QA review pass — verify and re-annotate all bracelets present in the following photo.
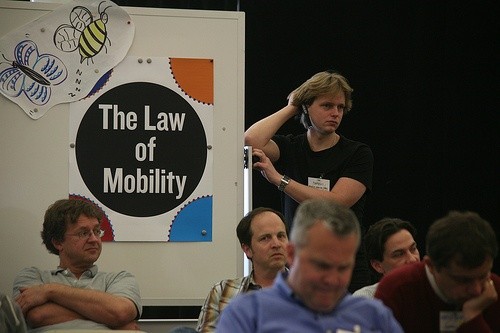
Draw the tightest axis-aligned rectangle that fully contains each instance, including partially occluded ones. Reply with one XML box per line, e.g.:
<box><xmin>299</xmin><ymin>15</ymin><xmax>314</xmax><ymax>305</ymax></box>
<box><xmin>278</xmin><ymin>175</ymin><xmax>291</xmax><ymax>191</ymax></box>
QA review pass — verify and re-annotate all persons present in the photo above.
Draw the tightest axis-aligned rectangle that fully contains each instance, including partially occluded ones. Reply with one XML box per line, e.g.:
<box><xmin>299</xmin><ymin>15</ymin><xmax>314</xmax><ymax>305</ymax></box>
<box><xmin>372</xmin><ymin>212</ymin><xmax>500</xmax><ymax>332</ymax></box>
<box><xmin>350</xmin><ymin>219</ymin><xmax>422</xmax><ymax>299</ymax></box>
<box><xmin>9</xmin><ymin>199</ymin><xmax>146</xmax><ymax>333</ymax></box>
<box><xmin>213</xmin><ymin>196</ymin><xmax>405</xmax><ymax>333</ymax></box>
<box><xmin>244</xmin><ymin>71</ymin><xmax>377</xmax><ymax>294</ymax></box>
<box><xmin>172</xmin><ymin>207</ymin><xmax>288</xmax><ymax>333</ymax></box>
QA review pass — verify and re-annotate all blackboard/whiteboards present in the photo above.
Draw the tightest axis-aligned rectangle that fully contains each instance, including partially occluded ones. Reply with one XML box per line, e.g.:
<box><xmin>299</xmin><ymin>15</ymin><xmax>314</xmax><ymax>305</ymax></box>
<box><xmin>0</xmin><ymin>0</ymin><xmax>253</xmax><ymax>307</ymax></box>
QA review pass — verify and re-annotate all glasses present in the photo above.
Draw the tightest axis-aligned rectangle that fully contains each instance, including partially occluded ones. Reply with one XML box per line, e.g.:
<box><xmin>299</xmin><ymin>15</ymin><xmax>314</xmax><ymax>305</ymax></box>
<box><xmin>64</xmin><ymin>229</ymin><xmax>105</xmax><ymax>241</ymax></box>
